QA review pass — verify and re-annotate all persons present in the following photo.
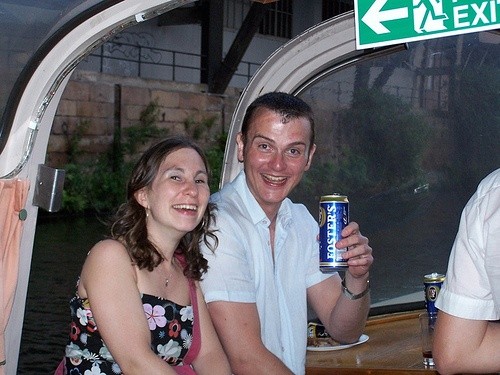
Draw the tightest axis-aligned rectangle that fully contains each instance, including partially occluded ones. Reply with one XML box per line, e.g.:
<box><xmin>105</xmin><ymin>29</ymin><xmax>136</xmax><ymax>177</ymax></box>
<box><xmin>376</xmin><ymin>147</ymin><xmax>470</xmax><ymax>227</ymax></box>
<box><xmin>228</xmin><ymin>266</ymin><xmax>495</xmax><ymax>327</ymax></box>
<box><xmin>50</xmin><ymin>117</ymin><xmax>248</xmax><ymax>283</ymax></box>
<box><xmin>63</xmin><ymin>136</ymin><xmax>236</xmax><ymax>375</ymax></box>
<box><xmin>193</xmin><ymin>93</ymin><xmax>373</xmax><ymax>375</ymax></box>
<box><xmin>432</xmin><ymin>168</ymin><xmax>500</xmax><ymax>374</ymax></box>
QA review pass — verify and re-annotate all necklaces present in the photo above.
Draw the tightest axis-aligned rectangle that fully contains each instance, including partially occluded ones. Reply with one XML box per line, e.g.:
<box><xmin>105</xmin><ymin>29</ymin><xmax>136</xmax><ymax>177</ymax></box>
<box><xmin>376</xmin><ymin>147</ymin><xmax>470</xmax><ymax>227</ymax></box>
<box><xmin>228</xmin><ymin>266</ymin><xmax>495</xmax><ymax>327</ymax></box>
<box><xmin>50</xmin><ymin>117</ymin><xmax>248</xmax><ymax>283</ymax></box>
<box><xmin>152</xmin><ymin>250</ymin><xmax>183</xmax><ymax>287</ymax></box>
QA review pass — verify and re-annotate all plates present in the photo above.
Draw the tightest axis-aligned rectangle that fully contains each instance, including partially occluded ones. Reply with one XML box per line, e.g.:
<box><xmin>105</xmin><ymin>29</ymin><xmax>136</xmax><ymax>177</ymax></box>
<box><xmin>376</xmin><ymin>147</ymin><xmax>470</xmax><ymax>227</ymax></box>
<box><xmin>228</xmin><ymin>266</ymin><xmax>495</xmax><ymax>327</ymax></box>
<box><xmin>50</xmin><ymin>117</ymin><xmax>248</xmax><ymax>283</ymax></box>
<box><xmin>306</xmin><ymin>333</ymin><xmax>369</xmax><ymax>352</ymax></box>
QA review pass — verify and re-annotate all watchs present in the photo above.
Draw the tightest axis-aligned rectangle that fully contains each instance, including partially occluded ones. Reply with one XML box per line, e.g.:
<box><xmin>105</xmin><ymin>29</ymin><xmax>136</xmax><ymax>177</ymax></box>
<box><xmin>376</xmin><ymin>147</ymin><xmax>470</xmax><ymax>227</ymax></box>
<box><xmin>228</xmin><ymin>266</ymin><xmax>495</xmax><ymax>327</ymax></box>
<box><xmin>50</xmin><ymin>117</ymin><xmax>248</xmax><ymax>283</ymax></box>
<box><xmin>340</xmin><ymin>277</ymin><xmax>371</xmax><ymax>300</ymax></box>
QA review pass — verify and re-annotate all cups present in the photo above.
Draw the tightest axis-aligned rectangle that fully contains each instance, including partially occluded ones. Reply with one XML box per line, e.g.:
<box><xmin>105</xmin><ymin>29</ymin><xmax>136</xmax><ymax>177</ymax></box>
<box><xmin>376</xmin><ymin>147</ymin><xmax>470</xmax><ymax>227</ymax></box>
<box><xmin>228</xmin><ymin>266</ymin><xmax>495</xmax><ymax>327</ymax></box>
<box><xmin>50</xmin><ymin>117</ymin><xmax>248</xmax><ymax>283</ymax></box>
<box><xmin>418</xmin><ymin>312</ymin><xmax>438</xmax><ymax>366</ymax></box>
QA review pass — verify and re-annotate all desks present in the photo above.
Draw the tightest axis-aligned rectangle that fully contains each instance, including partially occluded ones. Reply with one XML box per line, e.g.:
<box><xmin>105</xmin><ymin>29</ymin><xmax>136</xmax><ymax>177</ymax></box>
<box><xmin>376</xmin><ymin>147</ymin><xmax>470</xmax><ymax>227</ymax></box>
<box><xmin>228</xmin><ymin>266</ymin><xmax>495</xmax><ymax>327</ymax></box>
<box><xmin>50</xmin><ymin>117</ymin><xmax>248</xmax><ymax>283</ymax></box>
<box><xmin>304</xmin><ymin>309</ymin><xmax>440</xmax><ymax>375</ymax></box>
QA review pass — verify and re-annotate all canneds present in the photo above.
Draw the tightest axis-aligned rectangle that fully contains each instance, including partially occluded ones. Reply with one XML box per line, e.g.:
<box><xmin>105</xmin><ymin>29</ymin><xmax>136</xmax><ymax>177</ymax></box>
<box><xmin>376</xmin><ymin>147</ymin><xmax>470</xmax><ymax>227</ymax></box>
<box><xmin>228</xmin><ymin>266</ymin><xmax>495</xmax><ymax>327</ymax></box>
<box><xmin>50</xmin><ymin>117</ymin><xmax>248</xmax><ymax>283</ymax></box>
<box><xmin>424</xmin><ymin>272</ymin><xmax>446</xmax><ymax>327</ymax></box>
<box><xmin>318</xmin><ymin>192</ymin><xmax>349</xmax><ymax>272</ymax></box>
